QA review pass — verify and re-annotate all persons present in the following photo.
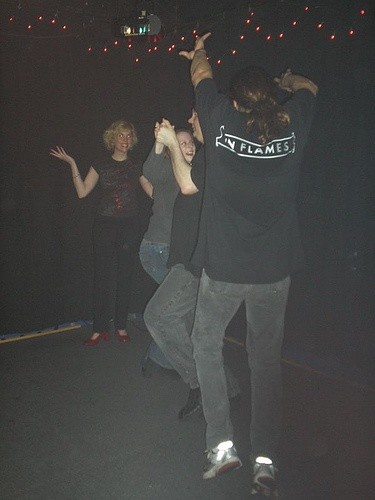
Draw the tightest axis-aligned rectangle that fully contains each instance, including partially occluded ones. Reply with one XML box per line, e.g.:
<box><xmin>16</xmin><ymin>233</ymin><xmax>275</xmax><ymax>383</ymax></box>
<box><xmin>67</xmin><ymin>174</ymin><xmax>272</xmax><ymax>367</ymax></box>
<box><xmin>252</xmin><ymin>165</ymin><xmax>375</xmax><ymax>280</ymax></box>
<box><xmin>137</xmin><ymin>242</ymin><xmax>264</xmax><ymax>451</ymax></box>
<box><xmin>50</xmin><ymin>98</ymin><xmax>241</xmax><ymax>420</ymax></box>
<box><xmin>173</xmin><ymin>26</ymin><xmax>319</xmax><ymax>498</ymax></box>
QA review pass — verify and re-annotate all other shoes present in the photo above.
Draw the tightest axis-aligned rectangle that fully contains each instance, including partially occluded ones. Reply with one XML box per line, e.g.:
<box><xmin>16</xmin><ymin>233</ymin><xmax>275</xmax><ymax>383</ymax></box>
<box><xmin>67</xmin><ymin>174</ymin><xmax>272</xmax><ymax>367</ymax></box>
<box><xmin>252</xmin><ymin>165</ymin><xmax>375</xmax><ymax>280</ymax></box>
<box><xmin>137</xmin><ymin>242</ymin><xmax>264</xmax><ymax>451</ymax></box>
<box><xmin>142</xmin><ymin>353</ymin><xmax>181</xmax><ymax>380</ymax></box>
<box><xmin>84</xmin><ymin>329</ymin><xmax>107</xmax><ymax>346</ymax></box>
<box><xmin>115</xmin><ymin>327</ymin><xmax>129</xmax><ymax>345</ymax></box>
<box><xmin>228</xmin><ymin>393</ymin><xmax>241</xmax><ymax>406</ymax></box>
<box><xmin>179</xmin><ymin>387</ymin><xmax>203</xmax><ymax>420</ymax></box>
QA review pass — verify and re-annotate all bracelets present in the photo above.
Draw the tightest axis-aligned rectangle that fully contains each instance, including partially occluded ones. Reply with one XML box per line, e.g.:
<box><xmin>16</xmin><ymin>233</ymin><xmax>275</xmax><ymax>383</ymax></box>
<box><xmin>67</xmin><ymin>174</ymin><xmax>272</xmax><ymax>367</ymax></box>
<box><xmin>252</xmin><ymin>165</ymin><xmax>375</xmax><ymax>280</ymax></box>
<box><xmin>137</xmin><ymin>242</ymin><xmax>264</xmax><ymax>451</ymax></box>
<box><xmin>71</xmin><ymin>173</ymin><xmax>79</xmax><ymax>180</ymax></box>
<box><xmin>169</xmin><ymin>148</ymin><xmax>180</xmax><ymax>155</ymax></box>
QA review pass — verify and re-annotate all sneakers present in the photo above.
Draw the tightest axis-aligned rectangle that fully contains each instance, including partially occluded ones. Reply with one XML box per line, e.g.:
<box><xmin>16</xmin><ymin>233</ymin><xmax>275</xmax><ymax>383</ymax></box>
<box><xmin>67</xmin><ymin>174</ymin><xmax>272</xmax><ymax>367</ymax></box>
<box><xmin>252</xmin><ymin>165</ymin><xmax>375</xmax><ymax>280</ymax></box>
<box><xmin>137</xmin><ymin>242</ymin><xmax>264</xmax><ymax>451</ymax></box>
<box><xmin>203</xmin><ymin>439</ymin><xmax>242</xmax><ymax>481</ymax></box>
<box><xmin>251</xmin><ymin>456</ymin><xmax>278</xmax><ymax>500</ymax></box>
